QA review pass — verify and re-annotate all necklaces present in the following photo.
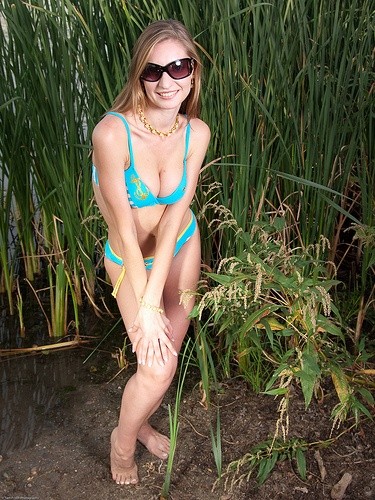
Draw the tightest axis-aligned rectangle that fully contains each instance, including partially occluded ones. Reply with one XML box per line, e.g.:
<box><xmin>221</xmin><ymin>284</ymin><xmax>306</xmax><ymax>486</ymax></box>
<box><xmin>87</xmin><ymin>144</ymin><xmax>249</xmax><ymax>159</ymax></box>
<box><xmin>136</xmin><ymin>103</ymin><xmax>180</xmax><ymax>137</ymax></box>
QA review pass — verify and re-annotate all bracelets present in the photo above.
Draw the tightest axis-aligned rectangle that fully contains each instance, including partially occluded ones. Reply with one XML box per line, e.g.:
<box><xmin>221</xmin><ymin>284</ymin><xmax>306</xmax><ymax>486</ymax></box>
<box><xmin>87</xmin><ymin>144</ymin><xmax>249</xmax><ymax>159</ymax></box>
<box><xmin>138</xmin><ymin>296</ymin><xmax>164</xmax><ymax>314</ymax></box>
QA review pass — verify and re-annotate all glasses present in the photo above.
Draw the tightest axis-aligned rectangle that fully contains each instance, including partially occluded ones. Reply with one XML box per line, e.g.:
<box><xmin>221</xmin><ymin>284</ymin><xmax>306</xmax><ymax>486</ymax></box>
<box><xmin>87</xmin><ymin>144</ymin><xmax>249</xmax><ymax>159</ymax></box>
<box><xmin>138</xmin><ymin>57</ymin><xmax>194</xmax><ymax>82</ymax></box>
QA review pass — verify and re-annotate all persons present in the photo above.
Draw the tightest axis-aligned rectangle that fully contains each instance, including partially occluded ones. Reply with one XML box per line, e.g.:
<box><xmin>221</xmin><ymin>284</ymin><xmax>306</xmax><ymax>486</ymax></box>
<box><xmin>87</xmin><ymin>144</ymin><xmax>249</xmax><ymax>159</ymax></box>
<box><xmin>92</xmin><ymin>21</ymin><xmax>210</xmax><ymax>484</ymax></box>
